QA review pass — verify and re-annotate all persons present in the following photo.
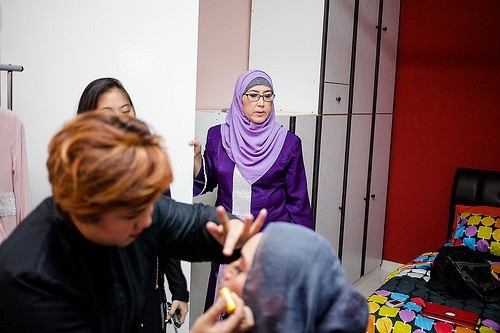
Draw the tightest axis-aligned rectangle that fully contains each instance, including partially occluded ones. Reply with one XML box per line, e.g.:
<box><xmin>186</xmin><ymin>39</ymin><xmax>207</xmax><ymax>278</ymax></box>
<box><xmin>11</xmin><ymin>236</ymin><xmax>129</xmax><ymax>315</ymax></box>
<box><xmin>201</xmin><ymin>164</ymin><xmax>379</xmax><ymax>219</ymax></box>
<box><xmin>221</xmin><ymin>221</ymin><xmax>369</xmax><ymax>333</ymax></box>
<box><xmin>0</xmin><ymin>77</ymin><xmax>269</xmax><ymax>333</ymax></box>
<box><xmin>189</xmin><ymin>68</ymin><xmax>315</xmax><ymax>321</ymax></box>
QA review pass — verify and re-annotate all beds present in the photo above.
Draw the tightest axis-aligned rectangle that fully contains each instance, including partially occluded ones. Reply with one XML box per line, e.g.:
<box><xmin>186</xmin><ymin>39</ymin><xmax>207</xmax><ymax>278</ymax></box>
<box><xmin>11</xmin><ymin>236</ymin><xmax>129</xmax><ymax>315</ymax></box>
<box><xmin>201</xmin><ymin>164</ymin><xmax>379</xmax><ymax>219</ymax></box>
<box><xmin>366</xmin><ymin>168</ymin><xmax>500</xmax><ymax>333</ymax></box>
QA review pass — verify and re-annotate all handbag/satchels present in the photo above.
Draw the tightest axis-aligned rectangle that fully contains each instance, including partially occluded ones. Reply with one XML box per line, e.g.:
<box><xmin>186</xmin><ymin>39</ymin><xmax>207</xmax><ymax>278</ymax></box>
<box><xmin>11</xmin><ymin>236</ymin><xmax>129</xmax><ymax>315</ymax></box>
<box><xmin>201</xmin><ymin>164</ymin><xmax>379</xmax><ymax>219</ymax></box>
<box><xmin>426</xmin><ymin>247</ymin><xmax>500</xmax><ymax>303</ymax></box>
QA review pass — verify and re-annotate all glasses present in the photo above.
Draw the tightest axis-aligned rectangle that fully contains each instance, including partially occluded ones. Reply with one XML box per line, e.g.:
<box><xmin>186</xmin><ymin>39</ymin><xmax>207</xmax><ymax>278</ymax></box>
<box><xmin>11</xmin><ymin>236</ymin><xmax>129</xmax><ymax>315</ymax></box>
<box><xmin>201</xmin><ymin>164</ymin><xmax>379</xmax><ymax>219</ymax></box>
<box><xmin>243</xmin><ymin>92</ymin><xmax>276</xmax><ymax>102</ymax></box>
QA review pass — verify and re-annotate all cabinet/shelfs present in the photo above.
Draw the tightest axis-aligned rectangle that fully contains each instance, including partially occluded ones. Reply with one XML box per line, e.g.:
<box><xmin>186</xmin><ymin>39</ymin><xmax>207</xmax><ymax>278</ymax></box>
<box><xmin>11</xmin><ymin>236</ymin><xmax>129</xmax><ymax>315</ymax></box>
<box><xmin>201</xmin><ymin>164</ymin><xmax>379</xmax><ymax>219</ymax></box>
<box><xmin>249</xmin><ymin>0</ymin><xmax>399</xmax><ymax>286</ymax></box>
